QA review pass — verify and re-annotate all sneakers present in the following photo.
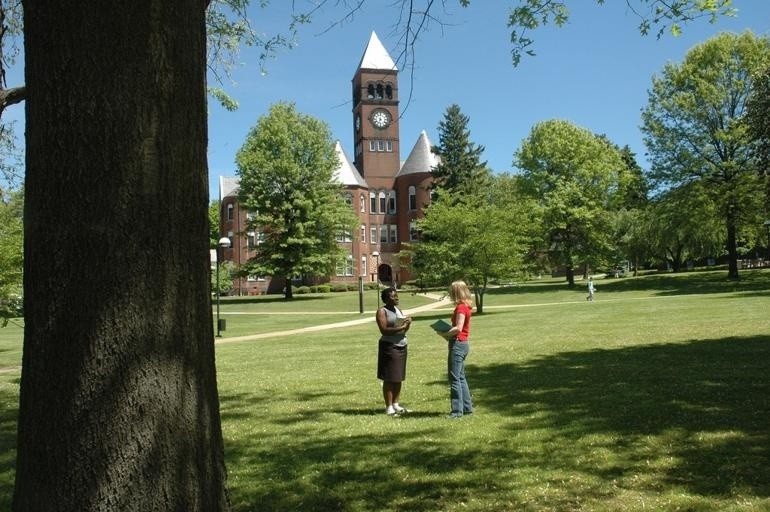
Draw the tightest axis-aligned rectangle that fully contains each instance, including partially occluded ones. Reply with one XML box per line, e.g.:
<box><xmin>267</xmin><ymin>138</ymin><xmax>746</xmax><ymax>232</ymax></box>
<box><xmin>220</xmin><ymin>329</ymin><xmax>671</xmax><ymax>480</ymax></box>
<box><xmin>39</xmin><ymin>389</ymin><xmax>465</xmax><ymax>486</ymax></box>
<box><xmin>393</xmin><ymin>406</ymin><xmax>407</xmax><ymax>414</ymax></box>
<box><xmin>386</xmin><ymin>410</ymin><xmax>399</xmax><ymax>417</ymax></box>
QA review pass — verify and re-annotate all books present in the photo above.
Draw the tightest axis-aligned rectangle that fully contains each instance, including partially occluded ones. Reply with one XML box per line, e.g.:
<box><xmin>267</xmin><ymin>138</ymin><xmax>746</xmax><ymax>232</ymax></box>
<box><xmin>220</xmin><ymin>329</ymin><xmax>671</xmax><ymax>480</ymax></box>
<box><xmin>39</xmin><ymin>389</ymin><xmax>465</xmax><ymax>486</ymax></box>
<box><xmin>432</xmin><ymin>319</ymin><xmax>456</xmax><ymax>337</ymax></box>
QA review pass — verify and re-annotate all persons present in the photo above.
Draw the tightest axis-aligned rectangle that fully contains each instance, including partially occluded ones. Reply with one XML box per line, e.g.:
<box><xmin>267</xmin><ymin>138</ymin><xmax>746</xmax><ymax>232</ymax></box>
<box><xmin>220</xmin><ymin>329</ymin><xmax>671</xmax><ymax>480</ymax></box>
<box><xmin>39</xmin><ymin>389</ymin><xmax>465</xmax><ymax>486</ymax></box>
<box><xmin>584</xmin><ymin>276</ymin><xmax>597</xmax><ymax>301</ymax></box>
<box><xmin>538</xmin><ymin>272</ymin><xmax>544</xmax><ymax>279</ymax></box>
<box><xmin>374</xmin><ymin>287</ymin><xmax>412</xmax><ymax>411</ymax></box>
<box><xmin>435</xmin><ymin>279</ymin><xmax>474</xmax><ymax>418</ymax></box>
<box><xmin>613</xmin><ymin>267</ymin><xmax>620</xmax><ymax>279</ymax></box>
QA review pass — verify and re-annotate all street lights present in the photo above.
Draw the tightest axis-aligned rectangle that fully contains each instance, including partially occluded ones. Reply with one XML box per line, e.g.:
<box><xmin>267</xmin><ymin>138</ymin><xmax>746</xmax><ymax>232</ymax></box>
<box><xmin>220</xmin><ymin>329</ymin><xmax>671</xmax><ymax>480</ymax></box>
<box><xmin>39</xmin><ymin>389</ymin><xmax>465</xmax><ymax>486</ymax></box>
<box><xmin>371</xmin><ymin>250</ymin><xmax>382</xmax><ymax>315</ymax></box>
<box><xmin>213</xmin><ymin>236</ymin><xmax>233</xmax><ymax>338</ymax></box>
